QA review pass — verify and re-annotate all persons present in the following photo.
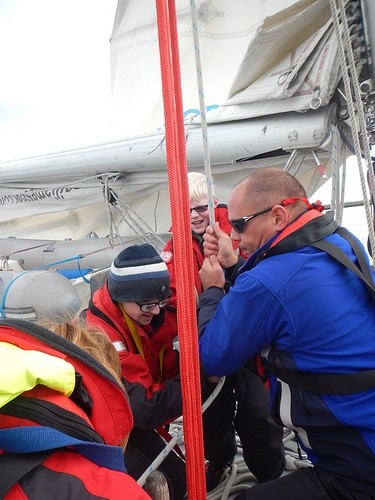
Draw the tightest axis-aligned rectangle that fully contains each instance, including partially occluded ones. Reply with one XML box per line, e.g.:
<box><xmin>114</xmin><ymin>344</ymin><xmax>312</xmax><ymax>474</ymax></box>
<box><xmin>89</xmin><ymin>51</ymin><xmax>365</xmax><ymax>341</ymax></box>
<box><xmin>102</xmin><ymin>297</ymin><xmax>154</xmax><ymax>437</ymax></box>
<box><xmin>159</xmin><ymin>169</ymin><xmax>375</xmax><ymax>498</ymax></box>
<box><xmin>1</xmin><ymin>243</ymin><xmax>188</xmax><ymax>500</ymax></box>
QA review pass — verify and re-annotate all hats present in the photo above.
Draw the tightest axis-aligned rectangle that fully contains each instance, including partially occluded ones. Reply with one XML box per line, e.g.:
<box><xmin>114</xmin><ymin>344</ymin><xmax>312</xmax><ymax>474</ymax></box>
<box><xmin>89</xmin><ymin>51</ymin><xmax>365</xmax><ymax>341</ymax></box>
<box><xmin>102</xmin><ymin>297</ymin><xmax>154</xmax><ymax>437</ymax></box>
<box><xmin>108</xmin><ymin>243</ymin><xmax>173</xmax><ymax>302</ymax></box>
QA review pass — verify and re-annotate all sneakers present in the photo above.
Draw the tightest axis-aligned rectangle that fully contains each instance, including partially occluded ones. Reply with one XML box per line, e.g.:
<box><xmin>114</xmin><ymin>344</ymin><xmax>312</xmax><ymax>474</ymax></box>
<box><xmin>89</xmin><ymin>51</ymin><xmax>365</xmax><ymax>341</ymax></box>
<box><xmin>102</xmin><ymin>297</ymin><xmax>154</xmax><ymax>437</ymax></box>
<box><xmin>205</xmin><ymin>454</ymin><xmax>235</xmax><ymax>492</ymax></box>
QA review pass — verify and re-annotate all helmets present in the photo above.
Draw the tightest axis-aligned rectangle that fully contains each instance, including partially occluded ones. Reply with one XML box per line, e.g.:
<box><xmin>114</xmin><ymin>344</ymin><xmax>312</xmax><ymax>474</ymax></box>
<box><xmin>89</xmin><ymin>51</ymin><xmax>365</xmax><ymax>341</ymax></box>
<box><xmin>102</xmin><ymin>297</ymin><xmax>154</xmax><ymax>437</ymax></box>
<box><xmin>0</xmin><ymin>268</ymin><xmax>82</xmax><ymax>331</ymax></box>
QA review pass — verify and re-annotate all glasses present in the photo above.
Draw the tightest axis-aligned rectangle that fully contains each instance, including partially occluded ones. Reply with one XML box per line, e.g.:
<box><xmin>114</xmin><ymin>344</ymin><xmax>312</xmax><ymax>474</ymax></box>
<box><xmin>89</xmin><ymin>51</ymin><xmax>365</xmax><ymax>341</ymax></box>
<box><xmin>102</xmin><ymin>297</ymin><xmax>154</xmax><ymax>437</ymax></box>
<box><xmin>190</xmin><ymin>204</ymin><xmax>209</xmax><ymax>214</ymax></box>
<box><xmin>228</xmin><ymin>202</ymin><xmax>284</xmax><ymax>233</ymax></box>
<box><xmin>135</xmin><ymin>297</ymin><xmax>170</xmax><ymax>313</ymax></box>
